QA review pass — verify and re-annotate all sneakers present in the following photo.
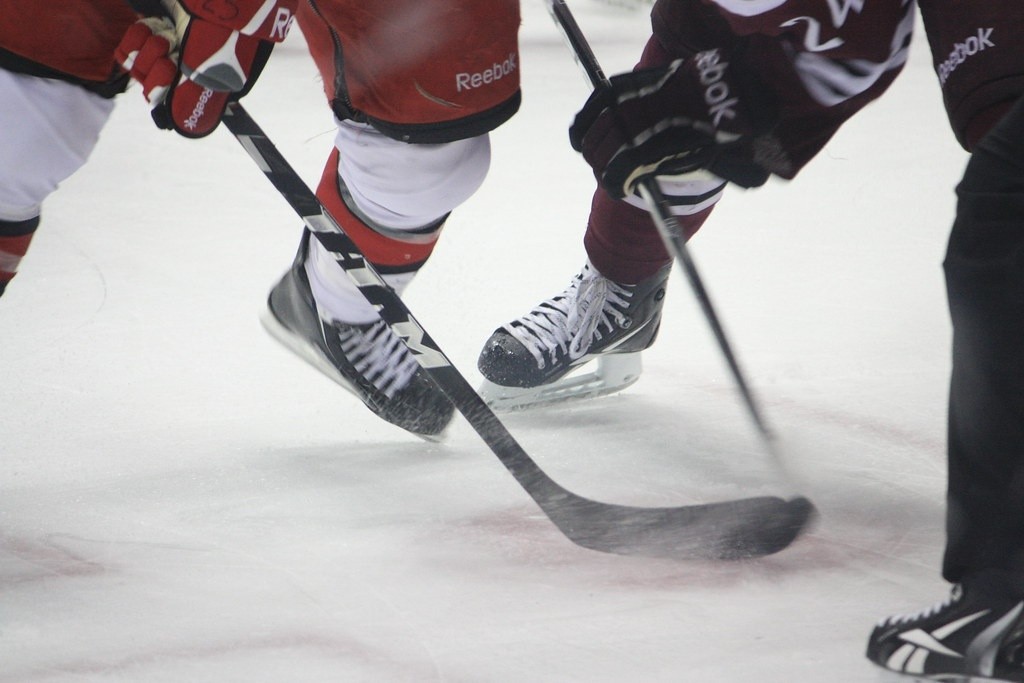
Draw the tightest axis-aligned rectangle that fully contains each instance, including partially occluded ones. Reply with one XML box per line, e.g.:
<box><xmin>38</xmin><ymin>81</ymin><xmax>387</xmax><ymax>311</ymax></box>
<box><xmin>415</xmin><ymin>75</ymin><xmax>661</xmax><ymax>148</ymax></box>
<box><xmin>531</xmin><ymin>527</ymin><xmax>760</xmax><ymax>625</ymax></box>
<box><xmin>864</xmin><ymin>580</ymin><xmax>1024</xmax><ymax>683</ymax></box>
<box><xmin>475</xmin><ymin>263</ymin><xmax>675</xmax><ymax>416</ymax></box>
<box><xmin>257</xmin><ymin>224</ymin><xmax>457</xmax><ymax>446</ymax></box>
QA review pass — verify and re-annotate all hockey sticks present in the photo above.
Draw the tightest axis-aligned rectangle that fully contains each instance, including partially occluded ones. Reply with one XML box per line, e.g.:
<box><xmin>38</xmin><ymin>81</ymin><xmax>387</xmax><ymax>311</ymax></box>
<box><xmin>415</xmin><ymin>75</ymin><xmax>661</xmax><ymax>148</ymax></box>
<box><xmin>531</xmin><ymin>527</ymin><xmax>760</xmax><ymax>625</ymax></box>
<box><xmin>542</xmin><ymin>1</ymin><xmax>942</xmax><ymax>528</ymax></box>
<box><xmin>218</xmin><ymin>97</ymin><xmax>820</xmax><ymax>566</ymax></box>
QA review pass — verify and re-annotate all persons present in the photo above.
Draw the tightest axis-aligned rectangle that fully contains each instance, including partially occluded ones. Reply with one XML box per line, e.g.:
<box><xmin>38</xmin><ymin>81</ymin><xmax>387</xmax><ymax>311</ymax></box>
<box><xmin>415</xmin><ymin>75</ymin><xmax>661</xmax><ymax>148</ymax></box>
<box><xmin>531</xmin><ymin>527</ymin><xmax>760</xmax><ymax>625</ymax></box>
<box><xmin>0</xmin><ymin>0</ymin><xmax>525</xmax><ymax>446</ymax></box>
<box><xmin>474</xmin><ymin>1</ymin><xmax>796</xmax><ymax>418</ymax></box>
<box><xmin>568</xmin><ymin>0</ymin><xmax>1024</xmax><ymax>683</ymax></box>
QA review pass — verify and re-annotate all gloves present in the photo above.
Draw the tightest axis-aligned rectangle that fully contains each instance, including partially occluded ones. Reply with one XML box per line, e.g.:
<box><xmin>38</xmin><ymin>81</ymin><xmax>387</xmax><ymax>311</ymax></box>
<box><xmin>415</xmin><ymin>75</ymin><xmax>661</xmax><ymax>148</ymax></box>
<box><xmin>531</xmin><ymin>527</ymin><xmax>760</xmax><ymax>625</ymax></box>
<box><xmin>112</xmin><ymin>0</ymin><xmax>299</xmax><ymax>134</ymax></box>
<box><xmin>569</xmin><ymin>33</ymin><xmax>790</xmax><ymax>207</ymax></box>
